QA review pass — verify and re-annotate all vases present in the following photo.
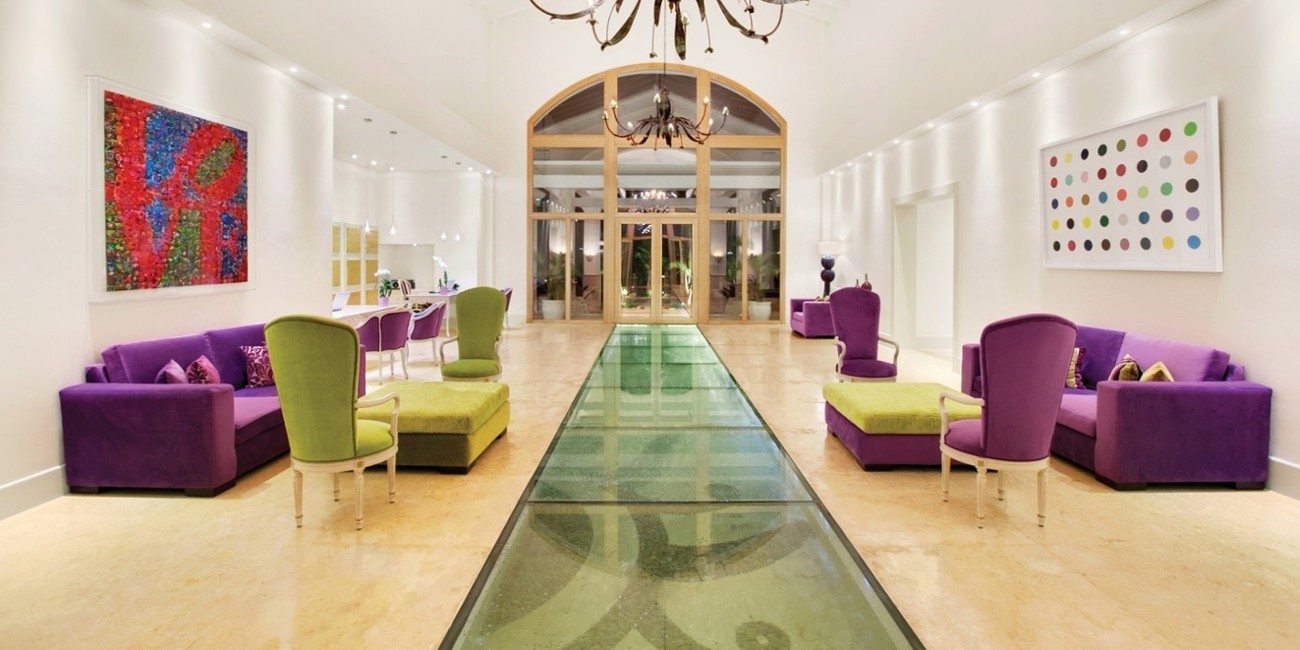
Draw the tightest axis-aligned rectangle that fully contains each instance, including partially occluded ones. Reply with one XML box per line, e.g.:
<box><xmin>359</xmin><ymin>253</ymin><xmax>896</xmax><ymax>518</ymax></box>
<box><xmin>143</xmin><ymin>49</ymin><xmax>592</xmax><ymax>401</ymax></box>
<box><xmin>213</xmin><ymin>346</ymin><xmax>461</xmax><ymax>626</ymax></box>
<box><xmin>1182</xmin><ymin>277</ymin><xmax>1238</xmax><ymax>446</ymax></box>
<box><xmin>440</xmin><ymin>286</ymin><xmax>449</xmax><ymax>295</ymax></box>
<box><xmin>378</xmin><ymin>297</ymin><xmax>389</xmax><ymax>307</ymax></box>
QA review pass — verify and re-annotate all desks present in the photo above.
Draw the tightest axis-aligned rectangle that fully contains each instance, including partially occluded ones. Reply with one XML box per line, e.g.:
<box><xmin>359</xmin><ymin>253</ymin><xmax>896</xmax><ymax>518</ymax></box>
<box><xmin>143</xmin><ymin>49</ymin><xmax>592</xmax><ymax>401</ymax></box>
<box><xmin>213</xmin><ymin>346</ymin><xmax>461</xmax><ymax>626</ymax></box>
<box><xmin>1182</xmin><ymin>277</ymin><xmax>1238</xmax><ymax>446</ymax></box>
<box><xmin>332</xmin><ymin>304</ymin><xmax>398</xmax><ymax>375</ymax></box>
<box><xmin>408</xmin><ymin>289</ymin><xmax>464</xmax><ymax>338</ymax></box>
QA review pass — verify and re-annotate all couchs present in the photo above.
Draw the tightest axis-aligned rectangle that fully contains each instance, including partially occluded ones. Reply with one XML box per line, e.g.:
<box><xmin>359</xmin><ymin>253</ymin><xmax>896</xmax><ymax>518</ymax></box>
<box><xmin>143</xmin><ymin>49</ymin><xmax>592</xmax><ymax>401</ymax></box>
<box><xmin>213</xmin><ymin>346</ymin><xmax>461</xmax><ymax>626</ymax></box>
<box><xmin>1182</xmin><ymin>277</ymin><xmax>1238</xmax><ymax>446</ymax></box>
<box><xmin>961</xmin><ymin>324</ymin><xmax>1272</xmax><ymax>490</ymax></box>
<box><xmin>789</xmin><ymin>297</ymin><xmax>835</xmax><ymax>340</ymax></box>
<box><xmin>57</xmin><ymin>323</ymin><xmax>365</xmax><ymax>499</ymax></box>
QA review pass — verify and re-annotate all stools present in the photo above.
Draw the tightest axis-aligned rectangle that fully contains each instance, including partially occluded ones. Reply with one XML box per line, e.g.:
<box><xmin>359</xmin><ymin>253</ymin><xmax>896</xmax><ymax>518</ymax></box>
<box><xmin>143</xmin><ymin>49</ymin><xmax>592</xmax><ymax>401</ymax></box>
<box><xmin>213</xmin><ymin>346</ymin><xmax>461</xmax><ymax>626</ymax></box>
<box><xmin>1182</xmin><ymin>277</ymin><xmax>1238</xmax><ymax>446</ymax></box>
<box><xmin>357</xmin><ymin>379</ymin><xmax>510</xmax><ymax>475</ymax></box>
<box><xmin>824</xmin><ymin>382</ymin><xmax>998</xmax><ymax>475</ymax></box>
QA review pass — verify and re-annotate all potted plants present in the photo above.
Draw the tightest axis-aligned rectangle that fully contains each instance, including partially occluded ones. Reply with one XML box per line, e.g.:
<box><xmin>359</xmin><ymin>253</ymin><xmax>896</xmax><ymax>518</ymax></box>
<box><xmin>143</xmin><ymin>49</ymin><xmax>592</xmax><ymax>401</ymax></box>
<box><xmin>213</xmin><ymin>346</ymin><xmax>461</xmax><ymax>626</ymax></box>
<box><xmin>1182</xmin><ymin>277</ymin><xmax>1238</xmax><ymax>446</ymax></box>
<box><xmin>746</xmin><ymin>252</ymin><xmax>779</xmax><ymax>319</ymax></box>
<box><xmin>534</xmin><ymin>252</ymin><xmax>577</xmax><ymax>320</ymax></box>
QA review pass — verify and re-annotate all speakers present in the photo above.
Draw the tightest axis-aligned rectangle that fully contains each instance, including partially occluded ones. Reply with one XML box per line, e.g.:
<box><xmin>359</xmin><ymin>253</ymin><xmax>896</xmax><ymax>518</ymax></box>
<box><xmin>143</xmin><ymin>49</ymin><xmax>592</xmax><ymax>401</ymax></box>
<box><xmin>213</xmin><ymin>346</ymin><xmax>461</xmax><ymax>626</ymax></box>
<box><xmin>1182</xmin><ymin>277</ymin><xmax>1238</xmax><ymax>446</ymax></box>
<box><xmin>390</xmin><ymin>279</ymin><xmax>399</xmax><ymax>288</ymax></box>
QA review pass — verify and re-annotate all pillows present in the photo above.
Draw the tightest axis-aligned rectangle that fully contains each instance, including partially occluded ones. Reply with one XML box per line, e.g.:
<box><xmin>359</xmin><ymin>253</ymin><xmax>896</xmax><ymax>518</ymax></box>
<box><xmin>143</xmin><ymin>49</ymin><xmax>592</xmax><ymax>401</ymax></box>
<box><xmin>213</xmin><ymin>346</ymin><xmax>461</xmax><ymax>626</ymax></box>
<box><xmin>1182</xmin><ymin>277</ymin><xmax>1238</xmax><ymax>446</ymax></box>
<box><xmin>154</xmin><ymin>360</ymin><xmax>190</xmax><ymax>385</ymax></box>
<box><xmin>185</xmin><ymin>356</ymin><xmax>220</xmax><ymax>384</ymax></box>
<box><xmin>238</xmin><ymin>345</ymin><xmax>275</xmax><ymax>387</ymax></box>
<box><xmin>1066</xmin><ymin>347</ymin><xmax>1175</xmax><ymax>385</ymax></box>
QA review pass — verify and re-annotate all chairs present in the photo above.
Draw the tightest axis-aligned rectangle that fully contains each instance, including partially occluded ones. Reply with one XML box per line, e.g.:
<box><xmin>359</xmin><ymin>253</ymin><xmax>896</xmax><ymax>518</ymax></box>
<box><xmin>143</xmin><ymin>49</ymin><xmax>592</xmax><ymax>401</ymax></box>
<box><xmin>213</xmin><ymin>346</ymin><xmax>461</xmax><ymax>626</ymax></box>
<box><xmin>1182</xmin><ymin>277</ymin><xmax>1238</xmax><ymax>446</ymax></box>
<box><xmin>263</xmin><ymin>314</ymin><xmax>400</xmax><ymax>530</ymax></box>
<box><xmin>398</xmin><ymin>280</ymin><xmax>432</xmax><ymax>312</ymax></box>
<box><xmin>440</xmin><ymin>287</ymin><xmax>502</xmax><ymax>383</ymax></box>
<box><xmin>938</xmin><ymin>312</ymin><xmax>1077</xmax><ymax>529</ymax></box>
<box><xmin>501</xmin><ymin>287</ymin><xmax>513</xmax><ymax>330</ymax></box>
<box><xmin>828</xmin><ymin>285</ymin><xmax>899</xmax><ymax>386</ymax></box>
<box><xmin>406</xmin><ymin>302</ymin><xmax>448</xmax><ymax>367</ymax></box>
<box><xmin>355</xmin><ymin>309</ymin><xmax>411</xmax><ymax>385</ymax></box>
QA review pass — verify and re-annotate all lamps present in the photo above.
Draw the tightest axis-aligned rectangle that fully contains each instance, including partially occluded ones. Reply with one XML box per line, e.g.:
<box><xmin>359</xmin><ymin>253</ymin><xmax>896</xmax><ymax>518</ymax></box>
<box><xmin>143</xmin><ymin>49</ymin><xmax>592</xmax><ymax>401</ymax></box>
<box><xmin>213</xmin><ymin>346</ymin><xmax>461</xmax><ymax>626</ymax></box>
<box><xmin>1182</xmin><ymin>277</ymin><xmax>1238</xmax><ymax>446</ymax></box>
<box><xmin>529</xmin><ymin>0</ymin><xmax>811</xmax><ymax>153</ymax></box>
<box><xmin>818</xmin><ymin>241</ymin><xmax>839</xmax><ymax>296</ymax></box>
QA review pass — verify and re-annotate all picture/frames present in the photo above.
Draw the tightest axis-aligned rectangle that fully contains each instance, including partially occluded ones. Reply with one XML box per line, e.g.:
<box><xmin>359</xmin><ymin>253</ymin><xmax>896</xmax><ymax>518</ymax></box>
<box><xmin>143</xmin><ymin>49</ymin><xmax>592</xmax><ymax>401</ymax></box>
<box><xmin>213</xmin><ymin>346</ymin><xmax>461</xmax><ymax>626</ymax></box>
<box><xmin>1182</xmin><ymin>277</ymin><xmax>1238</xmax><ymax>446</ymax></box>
<box><xmin>84</xmin><ymin>75</ymin><xmax>260</xmax><ymax>305</ymax></box>
<box><xmin>1036</xmin><ymin>97</ymin><xmax>1225</xmax><ymax>273</ymax></box>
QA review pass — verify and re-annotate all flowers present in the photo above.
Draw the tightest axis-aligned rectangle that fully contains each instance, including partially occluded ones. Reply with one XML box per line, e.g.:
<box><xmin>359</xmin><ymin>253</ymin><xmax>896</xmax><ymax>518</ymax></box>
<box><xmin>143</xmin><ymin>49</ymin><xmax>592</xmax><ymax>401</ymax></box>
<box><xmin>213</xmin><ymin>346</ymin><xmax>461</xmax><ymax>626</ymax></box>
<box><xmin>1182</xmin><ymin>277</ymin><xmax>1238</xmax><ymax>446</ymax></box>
<box><xmin>374</xmin><ymin>268</ymin><xmax>392</xmax><ymax>297</ymax></box>
<box><xmin>432</xmin><ymin>255</ymin><xmax>448</xmax><ymax>288</ymax></box>
